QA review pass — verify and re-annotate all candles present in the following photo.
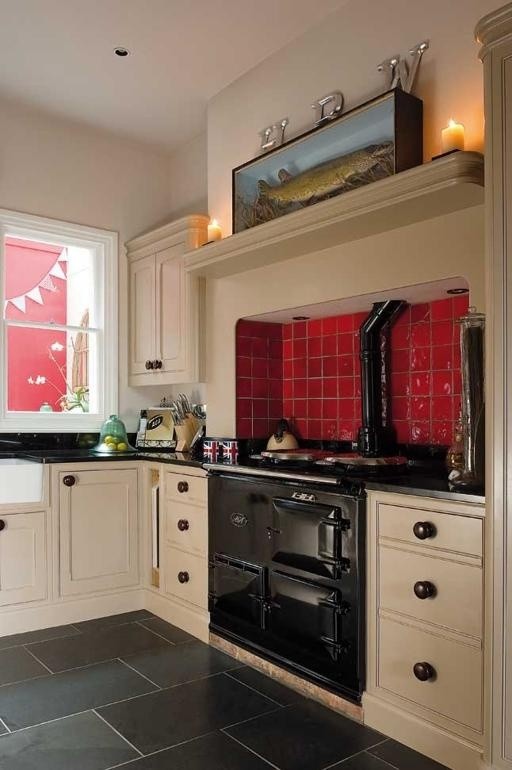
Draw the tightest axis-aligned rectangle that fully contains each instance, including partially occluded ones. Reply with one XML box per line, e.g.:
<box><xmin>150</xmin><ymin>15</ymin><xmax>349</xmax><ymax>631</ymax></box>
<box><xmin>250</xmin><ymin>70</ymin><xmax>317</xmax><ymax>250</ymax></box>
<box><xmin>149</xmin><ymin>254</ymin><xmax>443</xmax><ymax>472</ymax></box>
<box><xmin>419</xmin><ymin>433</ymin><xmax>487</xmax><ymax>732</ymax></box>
<box><xmin>206</xmin><ymin>218</ymin><xmax>222</xmax><ymax>240</ymax></box>
<box><xmin>438</xmin><ymin>116</ymin><xmax>468</xmax><ymax>153</ymax></box>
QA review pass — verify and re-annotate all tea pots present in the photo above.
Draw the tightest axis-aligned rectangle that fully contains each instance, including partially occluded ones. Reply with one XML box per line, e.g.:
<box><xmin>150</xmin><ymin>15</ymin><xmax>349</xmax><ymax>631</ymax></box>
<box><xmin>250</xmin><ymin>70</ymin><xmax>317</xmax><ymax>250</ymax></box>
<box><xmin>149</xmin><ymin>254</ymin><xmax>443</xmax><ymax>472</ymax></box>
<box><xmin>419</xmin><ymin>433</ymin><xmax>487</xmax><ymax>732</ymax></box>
<box><xmin>266</xmin><ymin>419</ymin><xmax>300</xmax><ymax>450</ymax></box>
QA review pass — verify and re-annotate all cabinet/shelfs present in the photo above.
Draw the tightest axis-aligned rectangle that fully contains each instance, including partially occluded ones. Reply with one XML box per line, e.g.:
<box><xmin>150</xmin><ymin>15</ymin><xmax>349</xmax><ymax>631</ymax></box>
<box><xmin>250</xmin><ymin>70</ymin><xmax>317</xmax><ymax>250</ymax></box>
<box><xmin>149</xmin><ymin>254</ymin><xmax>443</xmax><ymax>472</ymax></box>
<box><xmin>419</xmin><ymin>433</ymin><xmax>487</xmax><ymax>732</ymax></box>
<box><xmin>365</xmin><ymin>487</ymin><xmax>487</xmax><ymax>749</ymax></box>
<box><xmin>51</xmin><ymin>462</ymin><xmax>146</xmax><ymax>633</ymax></box>
<box><xmin>0</xmin><ymin>506</ymin><xmax>52</xmax><ymax>638</ymax></box>
<box><xmin>146</xmin><ymin>463</ymin><xmax>212</xmax><ymax>641</ymax></box>
<box><xmin>126</xmin><ymin>213</ymin><xmax>203</xmax><ymax>387</ymax></box>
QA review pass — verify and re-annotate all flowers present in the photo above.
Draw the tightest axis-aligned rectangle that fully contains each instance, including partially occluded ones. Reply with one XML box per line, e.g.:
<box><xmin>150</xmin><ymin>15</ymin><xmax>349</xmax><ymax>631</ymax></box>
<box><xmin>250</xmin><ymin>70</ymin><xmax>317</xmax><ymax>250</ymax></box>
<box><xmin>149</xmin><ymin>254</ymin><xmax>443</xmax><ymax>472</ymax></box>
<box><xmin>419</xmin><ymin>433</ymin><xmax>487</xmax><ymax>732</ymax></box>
<box><xmin>25</xmin><ymin>341</ymin><xmax>90</xmax><ymax>411</ymax></box>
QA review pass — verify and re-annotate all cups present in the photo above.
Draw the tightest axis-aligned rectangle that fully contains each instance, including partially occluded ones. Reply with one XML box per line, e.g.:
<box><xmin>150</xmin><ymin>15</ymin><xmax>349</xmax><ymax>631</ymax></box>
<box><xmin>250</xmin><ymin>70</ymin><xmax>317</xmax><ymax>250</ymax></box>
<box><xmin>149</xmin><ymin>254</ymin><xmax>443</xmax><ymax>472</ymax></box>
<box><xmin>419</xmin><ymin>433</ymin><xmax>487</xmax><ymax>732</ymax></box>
<box><xmin>203</xmin><ymin>440</ymin><xmax>220</xmax><ymax>459</ymax></box>
<box><xmin>222</xmin><ymin>441</ymin><xmax>240</xmax><ymax>458</ymax></box>
<box><xmin>203</xmin><ymin>458</ymin><xmax>239</xmax><ymax>466</ymax></box>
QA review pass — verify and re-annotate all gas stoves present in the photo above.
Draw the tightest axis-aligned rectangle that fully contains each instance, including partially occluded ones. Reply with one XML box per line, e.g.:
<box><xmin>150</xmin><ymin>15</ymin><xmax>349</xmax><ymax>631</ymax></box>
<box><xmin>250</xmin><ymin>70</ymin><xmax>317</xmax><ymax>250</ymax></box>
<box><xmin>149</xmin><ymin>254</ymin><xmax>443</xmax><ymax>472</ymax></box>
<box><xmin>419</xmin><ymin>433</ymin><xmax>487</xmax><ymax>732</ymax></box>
<box><xmin>239</xmin><ymin>450</ymin><xmax>433</xmax><ymax>476</ymax></box>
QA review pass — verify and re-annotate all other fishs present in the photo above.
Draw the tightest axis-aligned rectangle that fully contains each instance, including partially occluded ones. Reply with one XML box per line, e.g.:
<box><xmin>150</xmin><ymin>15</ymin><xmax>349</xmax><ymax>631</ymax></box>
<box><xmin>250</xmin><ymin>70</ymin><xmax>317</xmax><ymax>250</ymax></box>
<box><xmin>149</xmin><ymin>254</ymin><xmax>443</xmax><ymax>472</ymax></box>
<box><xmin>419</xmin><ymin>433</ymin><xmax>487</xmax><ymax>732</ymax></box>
<box><xmin>255</xmin><ymin>138</ymin><xmax>395</xmax><ymax>207</ymax></box>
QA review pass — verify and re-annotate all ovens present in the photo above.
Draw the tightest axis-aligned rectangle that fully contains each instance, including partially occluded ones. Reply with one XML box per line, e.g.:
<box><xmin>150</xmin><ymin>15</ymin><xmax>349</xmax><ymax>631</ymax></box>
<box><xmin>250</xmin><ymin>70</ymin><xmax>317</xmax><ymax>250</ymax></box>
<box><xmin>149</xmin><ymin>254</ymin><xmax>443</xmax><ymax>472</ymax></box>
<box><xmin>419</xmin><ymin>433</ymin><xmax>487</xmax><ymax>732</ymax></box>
<box><xmin>206</xmin><ymin>471</ymin><xmax>367</xmax><ymax>706</ymax></box>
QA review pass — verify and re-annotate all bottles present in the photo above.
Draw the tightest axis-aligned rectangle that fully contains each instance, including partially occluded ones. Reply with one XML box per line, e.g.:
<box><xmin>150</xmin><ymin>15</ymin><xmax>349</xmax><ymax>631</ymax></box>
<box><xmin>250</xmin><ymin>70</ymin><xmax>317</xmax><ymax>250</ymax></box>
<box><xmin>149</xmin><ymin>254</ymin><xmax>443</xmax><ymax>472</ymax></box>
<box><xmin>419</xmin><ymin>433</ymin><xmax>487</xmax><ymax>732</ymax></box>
<box><xmin>445</xmin><ymin>433</ymin><xmax>465</xmax><ymax>470</ymax></box>
<box><xmin>449</xmin><ymin>326</ymin><xmax>485</xmax><ymax>491</ymax></box>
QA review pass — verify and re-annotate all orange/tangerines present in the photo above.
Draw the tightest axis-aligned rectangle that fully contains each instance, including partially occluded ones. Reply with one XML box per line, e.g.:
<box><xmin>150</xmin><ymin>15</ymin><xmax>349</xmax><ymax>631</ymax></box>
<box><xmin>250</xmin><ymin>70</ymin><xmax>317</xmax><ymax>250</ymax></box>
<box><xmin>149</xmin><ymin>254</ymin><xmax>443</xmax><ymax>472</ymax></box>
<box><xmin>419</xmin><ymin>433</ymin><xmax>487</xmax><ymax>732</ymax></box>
<box><xmin>105</xmin><ymin>436</ymin><xmax>128</xmax><ymax>451</ymax></box>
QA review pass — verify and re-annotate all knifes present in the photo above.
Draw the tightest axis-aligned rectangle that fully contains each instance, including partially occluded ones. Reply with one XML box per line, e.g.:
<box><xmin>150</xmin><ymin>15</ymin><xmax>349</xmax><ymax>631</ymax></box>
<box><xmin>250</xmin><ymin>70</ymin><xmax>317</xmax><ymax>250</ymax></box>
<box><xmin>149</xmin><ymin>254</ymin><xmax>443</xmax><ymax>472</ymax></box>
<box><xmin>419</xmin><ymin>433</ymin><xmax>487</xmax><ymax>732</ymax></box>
<box><xmin>172</xmin><ymin>393</ymin><xmax>192</xmax><ymax>426</ymax></box>
